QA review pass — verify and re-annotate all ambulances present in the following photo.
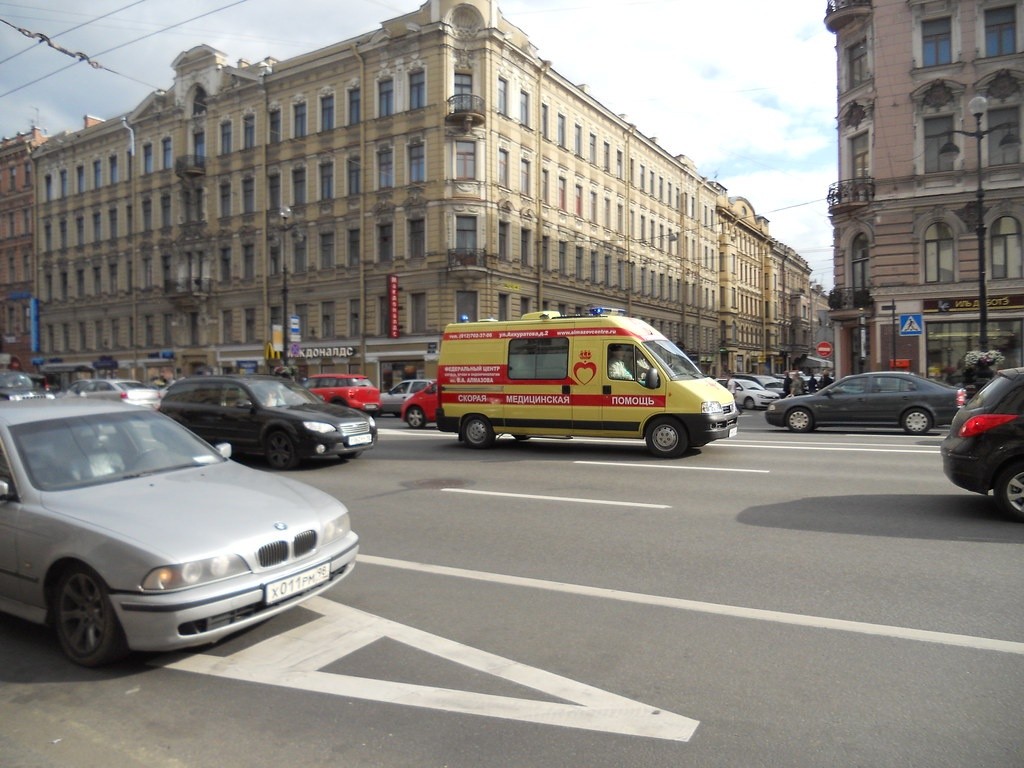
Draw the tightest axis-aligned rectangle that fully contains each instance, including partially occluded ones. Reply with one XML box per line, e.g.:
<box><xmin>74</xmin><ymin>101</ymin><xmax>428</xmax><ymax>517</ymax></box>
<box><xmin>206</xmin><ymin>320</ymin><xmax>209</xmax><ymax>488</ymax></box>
<box><xmin>430</xmin><ymin>306</ymin><xmax>741</xmax><ymax>459</ymax></box>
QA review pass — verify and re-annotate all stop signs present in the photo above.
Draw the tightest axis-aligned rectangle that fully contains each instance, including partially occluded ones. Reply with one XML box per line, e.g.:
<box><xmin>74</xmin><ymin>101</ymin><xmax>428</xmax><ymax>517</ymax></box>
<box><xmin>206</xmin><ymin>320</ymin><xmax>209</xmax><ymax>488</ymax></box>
<box><xmin>817</xmin><ymin>342</ymin><xmax>832</xmax><ymax>357</ymax></box>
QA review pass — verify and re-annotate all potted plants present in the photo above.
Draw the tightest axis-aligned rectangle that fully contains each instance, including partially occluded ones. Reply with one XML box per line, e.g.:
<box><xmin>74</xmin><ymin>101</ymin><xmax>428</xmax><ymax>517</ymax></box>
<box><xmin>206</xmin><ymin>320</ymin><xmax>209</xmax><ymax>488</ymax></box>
<box><xmin>965</xmin><ymin>350</ymin><xmax>1006</xmax><ymax>379</ymax></box>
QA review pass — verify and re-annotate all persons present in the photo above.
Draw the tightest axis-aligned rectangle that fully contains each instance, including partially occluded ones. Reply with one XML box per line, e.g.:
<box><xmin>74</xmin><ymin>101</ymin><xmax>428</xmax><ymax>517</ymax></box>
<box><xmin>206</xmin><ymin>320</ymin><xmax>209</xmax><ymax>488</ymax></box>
<box><xmin>795</xmin><ymin>371</ymin><xmax>806</xmax><ymax>389</ymax></box>
<box><xmin>263</xmin><ymin>386</ymin><xmax>285</xmax><ymax>409</ymax></box>
<box><xmin>725</xmin><ymin>369</ymin><xmax>733</xmax><ymax>393</ymax></box>
<box><xmin>807</xmin><ymin>373</ymin><xmax>819</xmax><ymax>393</ymax></box>
<box><xmin>635</xmin><ymin>347</ymin><xmax>666</xmax><ymax>383</ymax></box>
<box><xmin>847</xmin><ymin>379</ymin><xmax>867</xmax><ymax>394</ymax></box>
<box><xmin>872</xmin><ymin>377</ymin><xmax>881</xmax><ymax>394</ymax></box>
<box><xmin>782</xmin><ymin>369</ymin><xmax>794</xmax><ymax>396</ymax></box>
<box><xmin>789</xmin><ymin>373</ymin><xmax>805</xmax><ymax>396</ymax></box>
<box><xmin>399</xmin><ymin>385</ymin><xmax>408</xmax><ymax>392</ymax></box>
<box><xmin>820</xmin><ymin>368</ymin><xmax>834</xmax><ymax>389</ymax></box>
<box><xmin>610</xmin><ymin>343</ymin><xmax>639</xmax><ymax>383</ymax></box>
<box><xmin>725</xmin><ymin>374</ymin><xmax>744</xmax><ymax>416</ymax></box>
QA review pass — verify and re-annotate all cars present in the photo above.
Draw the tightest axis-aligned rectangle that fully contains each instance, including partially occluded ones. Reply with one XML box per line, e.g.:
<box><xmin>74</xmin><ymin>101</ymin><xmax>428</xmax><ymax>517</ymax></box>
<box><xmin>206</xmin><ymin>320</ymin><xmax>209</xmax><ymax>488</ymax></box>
<box><xmin>722</xmin><ymin>369</ymin><xmax>834</xmax><ymax>409</ymax></box>
<box><xmin>939</xmin><ymin>366</ymin><xmax>1024</xmax><ymax>518</ymax></box>
<box><xmin>0</xmin><ymin>399</ymin><xmax>367</xmax><ymax>670</ymax></box>
<box><xmin>154</xmin><ymin>375</ymin><xmax>381</xmax><ymax>468</ymax></box>
<box><xmin>300</xmin><ymin>374</ymin><xmax>382</xmax><ymax>418</ymax></box>
<box><xmin>765</xmin><ymin>371</ymin><xmax>968</xmax><ymax>436</ymax></box>
<box><xmin>379</xmin><ymin>377</ymin><xmax>437</xmax><ymax>416</ymax></box>
<box><xmin>0</xmin><ymin>368</ymin><xmax>172</xmax><ymax>412</ymax></box>
<box><xmin>401</xmin><ymin>381</ymin><xmax>438</xmax><ymax>430</ymax></box>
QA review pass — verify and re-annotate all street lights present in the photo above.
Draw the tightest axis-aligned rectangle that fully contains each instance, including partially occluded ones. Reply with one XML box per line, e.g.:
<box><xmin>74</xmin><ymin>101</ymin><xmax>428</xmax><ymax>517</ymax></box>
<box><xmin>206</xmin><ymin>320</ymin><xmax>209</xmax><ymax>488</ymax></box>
<box><xmin>266</xmin><ymin>207</ymin><xmax>305</xmax><ymax>378</ymax></box>
<box><xmin>937</xmin><ymin>95</ymin><xmax>1022</xmax><ymax>382</ymax></box>
